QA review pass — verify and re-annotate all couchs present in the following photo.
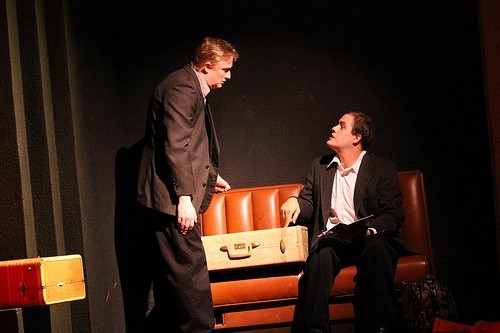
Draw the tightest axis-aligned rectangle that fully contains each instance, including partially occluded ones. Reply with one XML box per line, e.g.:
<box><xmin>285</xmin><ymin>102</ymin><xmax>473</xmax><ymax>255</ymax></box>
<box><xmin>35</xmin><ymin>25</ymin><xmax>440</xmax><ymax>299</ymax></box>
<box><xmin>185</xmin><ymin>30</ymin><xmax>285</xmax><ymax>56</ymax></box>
<box><xmin>198</xmin><ymin>170</ymin><xmax>435</xmax><ymax>331</ymax></box>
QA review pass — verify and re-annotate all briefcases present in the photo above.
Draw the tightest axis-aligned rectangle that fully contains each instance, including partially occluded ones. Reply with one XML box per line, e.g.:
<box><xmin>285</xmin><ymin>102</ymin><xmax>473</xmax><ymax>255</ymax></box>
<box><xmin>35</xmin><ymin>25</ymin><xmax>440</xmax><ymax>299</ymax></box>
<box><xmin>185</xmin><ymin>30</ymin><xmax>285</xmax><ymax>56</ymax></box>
<box><xmin>201</xmin><ymin>226</ymin><xmax>310</xmax><ymax>271</ymax></box>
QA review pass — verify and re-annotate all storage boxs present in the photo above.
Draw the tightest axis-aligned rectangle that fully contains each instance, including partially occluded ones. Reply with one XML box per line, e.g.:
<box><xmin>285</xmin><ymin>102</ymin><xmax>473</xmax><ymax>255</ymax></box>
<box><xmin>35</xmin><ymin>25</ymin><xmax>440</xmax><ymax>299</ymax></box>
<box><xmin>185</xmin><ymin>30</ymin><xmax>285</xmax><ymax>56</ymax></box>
<box><xmin>0</xmin><ymin>254</ymin><xmax>86</xmax><ymax>307</ymax></box>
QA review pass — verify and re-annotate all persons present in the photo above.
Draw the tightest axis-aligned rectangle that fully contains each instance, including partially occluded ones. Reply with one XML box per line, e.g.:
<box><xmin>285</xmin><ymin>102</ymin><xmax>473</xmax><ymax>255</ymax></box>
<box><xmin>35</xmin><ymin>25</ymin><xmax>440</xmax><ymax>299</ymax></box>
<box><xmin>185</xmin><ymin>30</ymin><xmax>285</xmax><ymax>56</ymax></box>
<box><xmin>135</xmin><ymin>32</ymin><xmax>239</xmax><ymax>332</ymax></box>
<box><xmin>279</xmin><ymin>109</ymin><xmax>404</xmax><ymax>333</ymax></box>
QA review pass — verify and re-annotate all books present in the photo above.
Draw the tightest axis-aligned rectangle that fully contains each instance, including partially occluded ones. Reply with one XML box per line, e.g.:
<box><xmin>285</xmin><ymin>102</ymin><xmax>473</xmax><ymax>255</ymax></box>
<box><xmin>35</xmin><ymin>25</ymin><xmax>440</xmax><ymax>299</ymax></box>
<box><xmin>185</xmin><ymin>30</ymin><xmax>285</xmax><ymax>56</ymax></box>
<box><xmin>316</xmin><ymin>214</ymin><xmax>373</xmax><ymax>233</ymax></box>
